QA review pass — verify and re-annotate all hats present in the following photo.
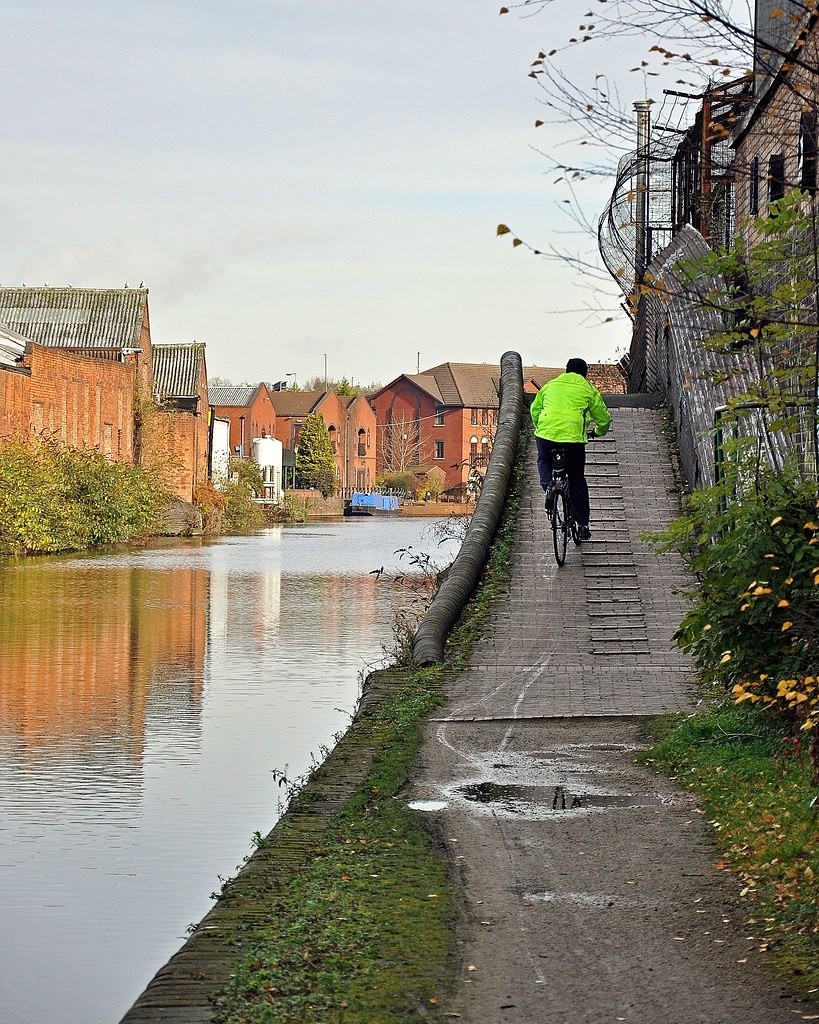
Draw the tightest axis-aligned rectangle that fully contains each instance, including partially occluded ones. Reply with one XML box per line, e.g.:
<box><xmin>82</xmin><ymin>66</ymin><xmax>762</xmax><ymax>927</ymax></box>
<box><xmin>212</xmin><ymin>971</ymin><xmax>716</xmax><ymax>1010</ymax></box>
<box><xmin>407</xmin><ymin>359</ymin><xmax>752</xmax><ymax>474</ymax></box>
<box><xmin>565</xmin><ymin>358</ymin><xmax>587</xmax><ymax>378</ymax></box>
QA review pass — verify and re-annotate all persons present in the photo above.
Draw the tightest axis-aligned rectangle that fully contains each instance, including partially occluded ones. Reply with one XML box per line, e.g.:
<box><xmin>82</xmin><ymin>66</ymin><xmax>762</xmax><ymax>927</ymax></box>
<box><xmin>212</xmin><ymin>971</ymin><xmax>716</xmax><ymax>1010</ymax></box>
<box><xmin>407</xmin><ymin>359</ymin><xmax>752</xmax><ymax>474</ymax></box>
<box><xmin>529</xmin><ymin>357</ymin><xmax>612</xmax><ymax>539</ymax></box>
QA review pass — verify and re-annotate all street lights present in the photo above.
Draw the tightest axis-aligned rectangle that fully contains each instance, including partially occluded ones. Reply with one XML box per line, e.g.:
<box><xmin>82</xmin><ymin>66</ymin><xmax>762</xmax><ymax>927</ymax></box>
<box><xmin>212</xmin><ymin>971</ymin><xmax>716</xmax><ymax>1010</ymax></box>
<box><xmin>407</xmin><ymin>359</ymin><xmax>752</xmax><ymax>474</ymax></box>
<box><xmin>417</xmin><ymin>352</ymin><xmax>420</xmax><ymax>373</ymax></box>
<box><xmin>234</xmin><ymin>415</ymin><xmax>245</xmax><ymax>459</ymax></box>
<box><xmin>286</xmin><ymin>372</ymin><xmax>297</xmax><ymax>392</ymax></box>
<box><xmin>324</xmin><ymin>354</ymin><xmax>327</xmax><ymax>393</ymax></box>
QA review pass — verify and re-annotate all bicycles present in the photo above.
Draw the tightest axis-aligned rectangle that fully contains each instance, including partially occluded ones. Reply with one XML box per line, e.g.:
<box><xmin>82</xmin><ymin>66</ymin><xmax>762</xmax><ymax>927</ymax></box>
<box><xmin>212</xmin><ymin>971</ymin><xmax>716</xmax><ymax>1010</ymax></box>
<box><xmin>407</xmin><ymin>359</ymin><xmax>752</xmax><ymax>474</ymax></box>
<box><xmin>544</xmin><ymin>427</ymin><xmax>595</xmax><ymax>567</ymax></box>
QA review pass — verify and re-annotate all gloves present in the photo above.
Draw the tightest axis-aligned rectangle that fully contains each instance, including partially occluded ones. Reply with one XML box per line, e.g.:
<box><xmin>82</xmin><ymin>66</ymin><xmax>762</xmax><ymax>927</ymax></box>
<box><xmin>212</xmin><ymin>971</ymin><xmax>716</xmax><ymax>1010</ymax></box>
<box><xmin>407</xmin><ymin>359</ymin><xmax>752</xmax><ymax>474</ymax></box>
<box><xmin>590</xmin><ymin>430</ymin><xmax>599</xmax><ymax>437</ymax></box>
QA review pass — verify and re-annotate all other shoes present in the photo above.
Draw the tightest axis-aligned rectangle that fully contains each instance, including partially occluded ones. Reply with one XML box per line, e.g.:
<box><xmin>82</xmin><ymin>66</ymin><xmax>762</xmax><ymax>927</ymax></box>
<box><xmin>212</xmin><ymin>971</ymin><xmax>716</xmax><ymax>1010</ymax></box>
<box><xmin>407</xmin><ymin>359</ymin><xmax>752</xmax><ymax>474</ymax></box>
<box><xmin>577</xmin><ymin>526</ymin><xmax>592</xmax><ymax>538</ymax></box>
<box><xmin>545</xmin><ymin>490</ymin><xmax>555</xmax><ymax>509</ymax></box>
